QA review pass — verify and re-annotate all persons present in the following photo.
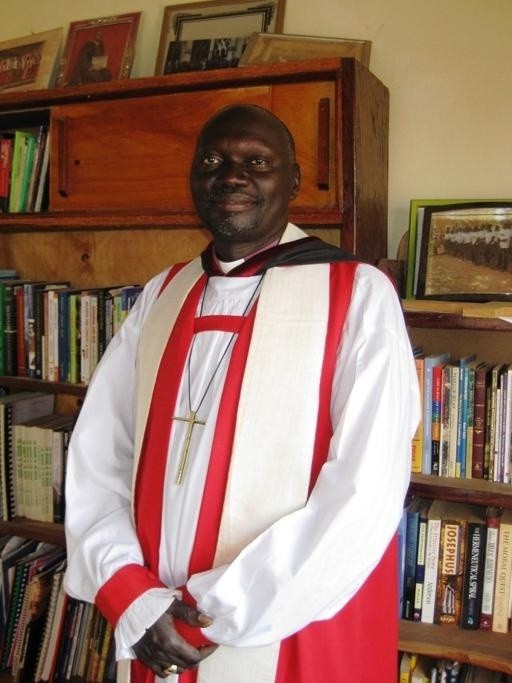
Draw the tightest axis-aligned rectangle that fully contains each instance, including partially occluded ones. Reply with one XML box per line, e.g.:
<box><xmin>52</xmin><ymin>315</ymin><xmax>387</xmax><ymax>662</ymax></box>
<box><xmin>69</xmin><ymin>29</ymin><xmax>113</xmax><ymax>84</ymax></box>
<box><xmin>431</xmin><ymin>220</ymin><xmax>511</xmax><ymax>270</ymax></box>
<box><xmin>59</xmin><ymin>103</ymin><xmax>423</xmax><ymax>682</ymax></box>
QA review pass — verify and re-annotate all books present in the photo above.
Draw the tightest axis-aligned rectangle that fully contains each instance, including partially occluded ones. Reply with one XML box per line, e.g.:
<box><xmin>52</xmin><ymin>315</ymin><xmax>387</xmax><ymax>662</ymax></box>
<box><xmin>397</xmin><ymin>343</ymin><xmax>511</xmax><ymax>680</ymax></box>
<box><xmin>1</xmin><ymin>123</ymin><xmax>50</xmax><ymax>212</ymax></box>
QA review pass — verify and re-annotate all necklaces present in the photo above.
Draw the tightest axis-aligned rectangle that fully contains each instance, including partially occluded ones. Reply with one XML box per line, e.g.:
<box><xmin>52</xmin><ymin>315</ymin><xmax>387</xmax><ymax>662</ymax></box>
<box><xmin>172</xmin><ymin>274</ymin><xmax>264</xmax><ymax>482</ymax></box>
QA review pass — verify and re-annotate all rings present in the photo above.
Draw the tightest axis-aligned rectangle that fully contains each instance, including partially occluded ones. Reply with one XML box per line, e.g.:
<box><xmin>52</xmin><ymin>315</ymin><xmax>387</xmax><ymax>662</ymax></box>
<box><xmin>163</xmin><ymin>664</ymin><xmax>178</xmax><ymax>676</ymax></box>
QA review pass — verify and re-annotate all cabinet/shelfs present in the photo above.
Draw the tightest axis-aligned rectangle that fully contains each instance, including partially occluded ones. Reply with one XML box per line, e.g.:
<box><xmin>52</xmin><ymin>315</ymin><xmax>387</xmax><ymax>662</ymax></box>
<box><xmin>393</xmin><ymin>297</ymin><xmax>512</xmax><ymax>683</ymax></box>
<box><xmin>2</xmin><ymin>57</ymin><xmax>389</xmax><ymax>683</ymax></box>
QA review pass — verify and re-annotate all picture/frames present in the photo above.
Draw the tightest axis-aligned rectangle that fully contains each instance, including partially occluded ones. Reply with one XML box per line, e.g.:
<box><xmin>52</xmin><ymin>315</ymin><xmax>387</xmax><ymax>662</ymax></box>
<box><xmin>153</xmin><ymin>0</ymin><xmax>284</xmax><ymax>75</ymax></box>
<box><xmin>0</xmin><ymin>11</ymin><xmax>142</xmax><ymax>93</ymax></box>
<box><xmin>416</xmin><ymin>200</ymin><xmax>512</xmax><ymax>303</ymax></box>
